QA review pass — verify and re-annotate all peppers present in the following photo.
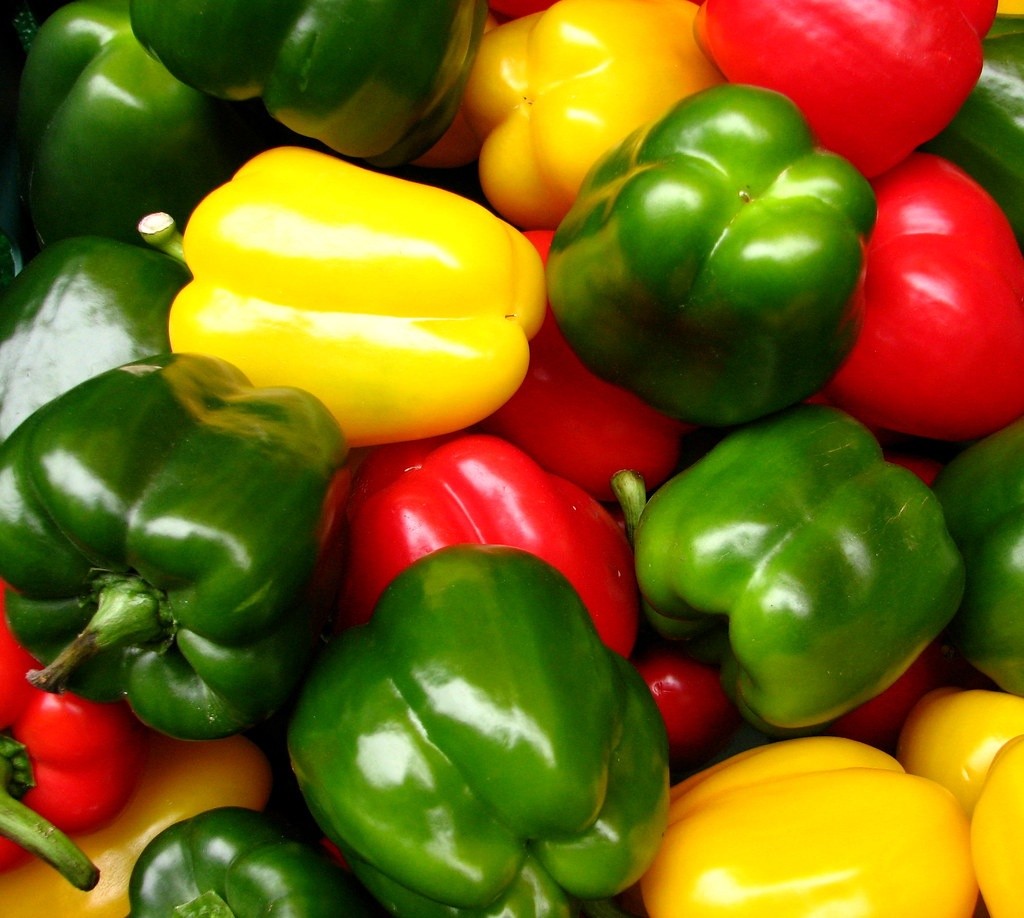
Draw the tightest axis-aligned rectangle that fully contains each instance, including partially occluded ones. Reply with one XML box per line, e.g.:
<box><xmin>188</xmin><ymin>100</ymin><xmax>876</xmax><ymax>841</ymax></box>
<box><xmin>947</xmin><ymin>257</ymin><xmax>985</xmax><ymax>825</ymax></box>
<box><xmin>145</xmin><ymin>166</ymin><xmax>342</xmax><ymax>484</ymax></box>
<box><xmin>0</xmin><ymin>0</ymin><xmax>1024</xmax><ymax>918</ymax></box>
